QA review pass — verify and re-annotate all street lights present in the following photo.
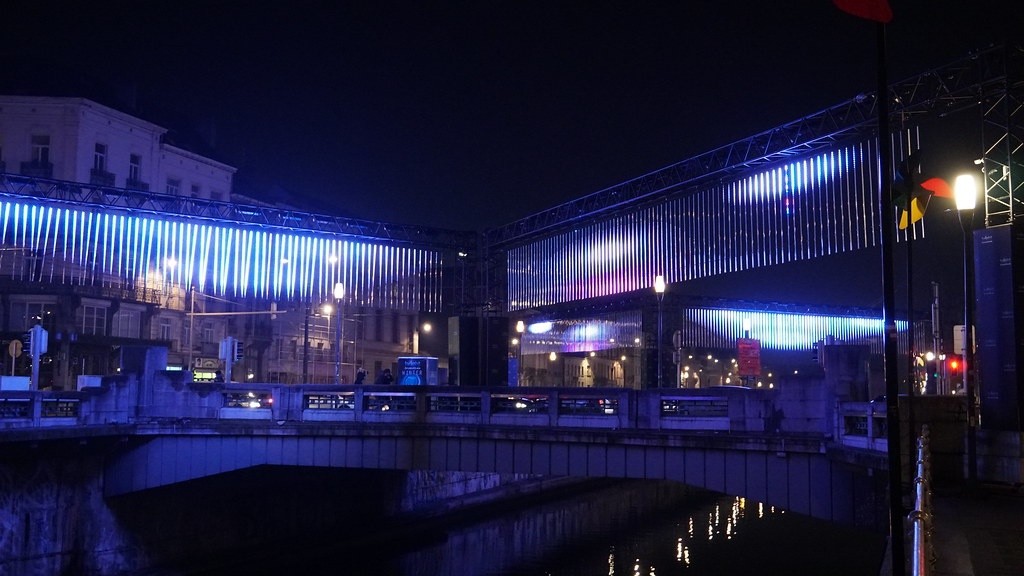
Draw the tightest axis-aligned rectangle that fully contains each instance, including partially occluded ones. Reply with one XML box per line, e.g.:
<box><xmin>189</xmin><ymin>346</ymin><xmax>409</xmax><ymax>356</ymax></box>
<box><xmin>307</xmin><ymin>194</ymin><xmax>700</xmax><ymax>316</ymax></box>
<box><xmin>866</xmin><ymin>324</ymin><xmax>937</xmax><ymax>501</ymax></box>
<box><xmin>954</xmin><ymin>172</ymin><xmax>978</xmax><ymax>484</ymax></box>
<box><xmin>332</xmin><ymin>282</ymin><xmax>345</xmax><ymax>384</ymax></box>
<box><xmin>653</xmin><ymin>274</ymin><xmax>666</xmax><ymax>388</ymax></box>
<box><xmin>515</xmin><ymin>320</ymin><xmax>526</xmax><ymax>386</ymax></box>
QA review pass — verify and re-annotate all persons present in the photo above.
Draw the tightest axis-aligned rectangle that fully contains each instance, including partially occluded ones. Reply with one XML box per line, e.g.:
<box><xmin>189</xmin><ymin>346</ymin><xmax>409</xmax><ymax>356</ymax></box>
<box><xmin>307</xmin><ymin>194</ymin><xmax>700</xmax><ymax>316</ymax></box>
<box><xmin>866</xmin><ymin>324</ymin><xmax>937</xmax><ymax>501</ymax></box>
<box><xmin>353</xmin><ymin>370</ymin><xmax>365</xmax><ymax>413</ymax></box>
<box><xmin>376</xmin><ymin>369</ymin><xmax>393</xmax><ymax>411</ymax></box>
<box><xmin>213</xmin><ymin>371</ymin><xmax>227</xmax><ymax>408</ymax></box>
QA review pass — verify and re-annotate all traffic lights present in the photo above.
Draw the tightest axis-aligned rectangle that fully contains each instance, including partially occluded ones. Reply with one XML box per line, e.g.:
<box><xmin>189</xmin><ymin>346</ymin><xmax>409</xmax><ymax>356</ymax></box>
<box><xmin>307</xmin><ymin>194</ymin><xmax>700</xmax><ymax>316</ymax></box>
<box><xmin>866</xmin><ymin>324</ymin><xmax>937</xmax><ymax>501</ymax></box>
<box><xmin>237</xmin><ymin>341</ymin><xmax>244</xmax><ymax>360</ymax></box>
